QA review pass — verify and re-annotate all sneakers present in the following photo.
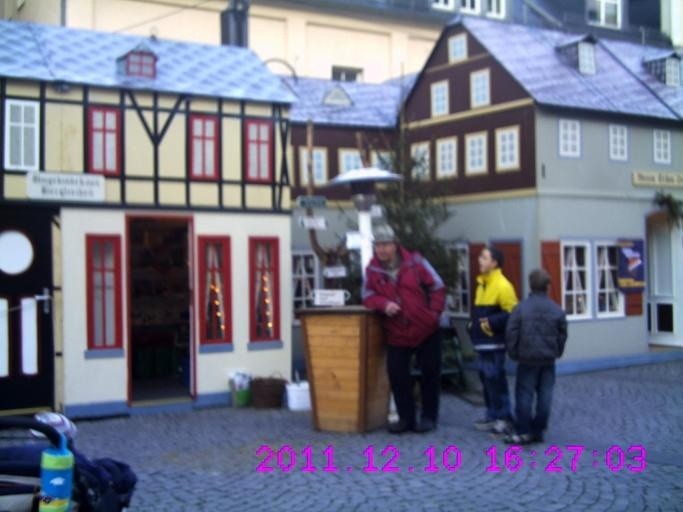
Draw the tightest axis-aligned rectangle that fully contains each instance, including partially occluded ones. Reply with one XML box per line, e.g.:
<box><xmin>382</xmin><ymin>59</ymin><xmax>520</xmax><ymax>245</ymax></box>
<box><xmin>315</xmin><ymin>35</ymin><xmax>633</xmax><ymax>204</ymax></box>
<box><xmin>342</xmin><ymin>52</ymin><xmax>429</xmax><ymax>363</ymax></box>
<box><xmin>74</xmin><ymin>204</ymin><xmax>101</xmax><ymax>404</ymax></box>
<box><xmin>493</xmin><ymin>417</ymin><xmax>512</xmax><ymax>433</ymax></box>
<box><xmin>503</xmin><ymin>433</ymin><xmax>532</xmax><ymax>445</ymax></box>
<box><xmin>471</xmin><ymin>419</ymin><xmax>494</xmax><ymax>432</ymax></box>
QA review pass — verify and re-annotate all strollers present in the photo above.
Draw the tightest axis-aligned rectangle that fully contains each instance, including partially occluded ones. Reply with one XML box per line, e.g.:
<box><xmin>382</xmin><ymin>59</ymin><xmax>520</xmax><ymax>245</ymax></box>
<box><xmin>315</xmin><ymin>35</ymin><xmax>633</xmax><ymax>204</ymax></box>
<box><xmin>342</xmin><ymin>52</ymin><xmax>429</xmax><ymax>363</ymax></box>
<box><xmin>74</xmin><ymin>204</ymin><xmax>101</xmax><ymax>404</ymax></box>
<box><xmin>0</xmin><ymin>409</ymin><xmax>138</xmax><ymax>512</ymax></box>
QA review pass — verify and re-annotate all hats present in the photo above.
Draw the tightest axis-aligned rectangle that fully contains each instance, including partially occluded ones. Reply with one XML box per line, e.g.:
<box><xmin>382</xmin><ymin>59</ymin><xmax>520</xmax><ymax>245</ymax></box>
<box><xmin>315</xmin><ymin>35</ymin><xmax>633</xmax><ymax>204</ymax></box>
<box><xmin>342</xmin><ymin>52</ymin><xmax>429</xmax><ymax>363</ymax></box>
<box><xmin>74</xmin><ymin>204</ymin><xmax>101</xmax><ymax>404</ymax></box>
<box><xmin>529</xmin><ymin>269</ymin><xmax>550</xmax><ymax>288</ymax></box>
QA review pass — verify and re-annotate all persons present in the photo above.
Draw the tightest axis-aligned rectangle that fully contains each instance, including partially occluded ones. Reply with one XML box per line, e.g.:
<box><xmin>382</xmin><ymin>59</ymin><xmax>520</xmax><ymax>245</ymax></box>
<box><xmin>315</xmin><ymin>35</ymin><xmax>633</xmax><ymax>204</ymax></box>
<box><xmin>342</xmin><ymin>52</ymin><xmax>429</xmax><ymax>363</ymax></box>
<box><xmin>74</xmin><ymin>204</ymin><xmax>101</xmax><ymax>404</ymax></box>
<box><xmin>504</xmin><ymin>268</ymin><xmax>567</xmax><ymax>445</ymax></box>
<box><xmin>359</xmin><ymin>226</ymin><xmax>446</xmax><ymax>434</ymax></box>
<box><xmin>466</xmin><ymin>247</ymin><xmax>519</xmax><ymax>433</ymax></box>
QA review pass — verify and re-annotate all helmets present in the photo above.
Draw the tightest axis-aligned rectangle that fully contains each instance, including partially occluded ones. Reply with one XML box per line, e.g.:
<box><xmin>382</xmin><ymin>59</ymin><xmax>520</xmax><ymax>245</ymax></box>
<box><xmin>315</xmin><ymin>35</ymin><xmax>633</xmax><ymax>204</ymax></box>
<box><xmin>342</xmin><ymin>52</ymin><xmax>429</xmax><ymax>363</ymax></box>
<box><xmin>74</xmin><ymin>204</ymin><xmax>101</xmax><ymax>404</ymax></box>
<box><xmin>371</xmin><ymin>224</ymin><xmax>395</xmax><ymax>243</ymax></box>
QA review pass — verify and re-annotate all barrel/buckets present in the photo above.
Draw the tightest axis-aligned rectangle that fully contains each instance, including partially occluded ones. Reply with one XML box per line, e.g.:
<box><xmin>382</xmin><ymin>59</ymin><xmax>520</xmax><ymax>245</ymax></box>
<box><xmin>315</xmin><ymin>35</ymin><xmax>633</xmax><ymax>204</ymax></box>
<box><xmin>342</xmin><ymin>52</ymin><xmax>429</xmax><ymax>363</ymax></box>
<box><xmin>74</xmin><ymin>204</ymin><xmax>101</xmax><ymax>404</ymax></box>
<box><xmin>250</xmin><ymin>376</ymin><xmax>284</xmax><ymax>409</ymax></box>
<box><xmin>284</xmin><ymin>381</ymin><xmax>312</xmax><ymax>411</ymax></box>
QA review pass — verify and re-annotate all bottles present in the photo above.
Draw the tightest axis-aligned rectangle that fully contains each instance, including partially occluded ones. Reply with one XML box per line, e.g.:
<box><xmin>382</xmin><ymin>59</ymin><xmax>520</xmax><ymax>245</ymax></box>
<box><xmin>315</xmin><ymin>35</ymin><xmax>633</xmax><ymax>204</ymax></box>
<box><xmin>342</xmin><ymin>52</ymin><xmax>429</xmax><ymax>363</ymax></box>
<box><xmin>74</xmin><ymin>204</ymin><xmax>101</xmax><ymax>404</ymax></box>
<box><xmin>39</xmin><ymin>434</ymin><xmax>74</xmax><ymax>512</ymax></box>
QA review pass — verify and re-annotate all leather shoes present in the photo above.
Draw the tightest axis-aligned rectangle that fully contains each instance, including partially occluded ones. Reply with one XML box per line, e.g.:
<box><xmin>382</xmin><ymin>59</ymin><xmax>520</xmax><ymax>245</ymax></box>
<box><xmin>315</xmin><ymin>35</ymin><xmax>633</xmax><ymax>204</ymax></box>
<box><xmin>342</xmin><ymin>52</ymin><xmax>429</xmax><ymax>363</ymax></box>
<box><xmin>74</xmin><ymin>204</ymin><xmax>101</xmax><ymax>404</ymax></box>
<box><xmin>419</xmin><ymin>418</ymin><xmax>435</xmax><ymax>432</ymax></box>
<box><xmin>389</xmin><ymin>415</ymin><xmax>415</xmax><ymax>433</ymax></box>
